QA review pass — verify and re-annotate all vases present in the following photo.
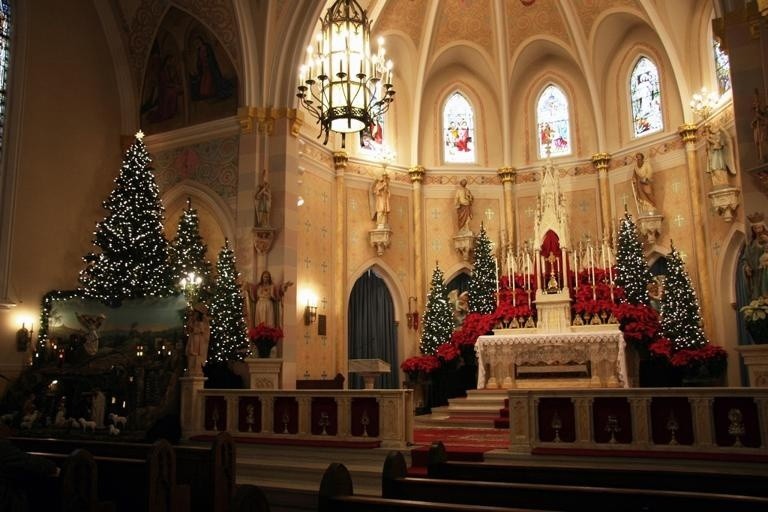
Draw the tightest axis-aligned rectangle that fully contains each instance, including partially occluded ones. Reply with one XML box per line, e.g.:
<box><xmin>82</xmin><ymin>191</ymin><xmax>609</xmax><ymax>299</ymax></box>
<box><xmin>255</xmin><ymin>344</ymin><xmax>280</xmax><ymax>361</ymax></box>
<box><xmin>744</xmin><ymin>321</ymin><xmax>767</xmax><ymax>345</ymax></box>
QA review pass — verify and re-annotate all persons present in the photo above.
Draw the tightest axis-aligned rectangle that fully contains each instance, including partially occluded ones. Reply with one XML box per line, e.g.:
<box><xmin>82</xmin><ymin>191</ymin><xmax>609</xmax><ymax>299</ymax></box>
<box><xmin>628</xmin><ymin>152</ymin><xmax>656</xmax><ymax>214</ymax></box>
<box><xmin>375</xmin><ymin>172</ymin><xmax>392</xmax><ymax>221</ymax></box>
<box><xmin>254</xmin><ymin>176</ymin><xmax>272</xmax><ymax>224</ymax></box>
<box><xmin>253</xmin><ymin>269</ymin><xmax>276</xmax><ymax>327</ymax></box>
<box><xmin>454</xmin><ymin>178</ymin><xmax>475</xmax><ymax>235</ymax></box>
<box><xmin>703</xmin><ymin>120</ymin><xmax>730</xmax><ymax>188</ymax></box>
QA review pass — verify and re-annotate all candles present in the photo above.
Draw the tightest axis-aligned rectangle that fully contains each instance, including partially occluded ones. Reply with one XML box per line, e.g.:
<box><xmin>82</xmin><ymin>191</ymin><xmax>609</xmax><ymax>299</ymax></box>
<box><xmin>492</xmin><ymin>244</ymin><xmax>613</xmax><ymax>290</ymax></box>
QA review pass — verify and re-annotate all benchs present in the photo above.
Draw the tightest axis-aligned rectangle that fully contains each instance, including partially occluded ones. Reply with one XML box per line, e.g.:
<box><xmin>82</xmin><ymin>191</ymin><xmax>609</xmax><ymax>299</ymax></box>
<box><xmin>317</xmin><ymin>440</ymin><xmax>768</xmax><ymax>512</ymax></box>
<box><xmin>0</xmin><ymin>431</ymin><xmax>237</xmax><ymax>511</ymax></box>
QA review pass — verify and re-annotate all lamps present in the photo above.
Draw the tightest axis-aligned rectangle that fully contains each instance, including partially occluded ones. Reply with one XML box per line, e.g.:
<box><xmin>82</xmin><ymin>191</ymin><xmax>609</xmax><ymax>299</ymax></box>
<box><xmin>689</xmin><ymin>87</ymin><xmax>716</xmax><ymax>118</ymax></box>
<box><xmin>293</xmin><ymin>0</ymin><xmax>394</xmax><ymax>149</ymax></box>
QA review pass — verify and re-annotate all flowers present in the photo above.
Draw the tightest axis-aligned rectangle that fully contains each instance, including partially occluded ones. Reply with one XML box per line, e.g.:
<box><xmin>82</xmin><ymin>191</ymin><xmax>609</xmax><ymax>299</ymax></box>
<box><xmin>248</xmin><ymin>321</ymin><xmax>287</xmax><ymax>342</ymax></box>
<box><xmin>738</xmin><ymin>297</ymin><xmax>767</xmax><ymax>322</ymax></box>
<box><xmin>400</xmin><ymin>269</ymin><xmax>727</xmax><ymax>383</ymax></box>
